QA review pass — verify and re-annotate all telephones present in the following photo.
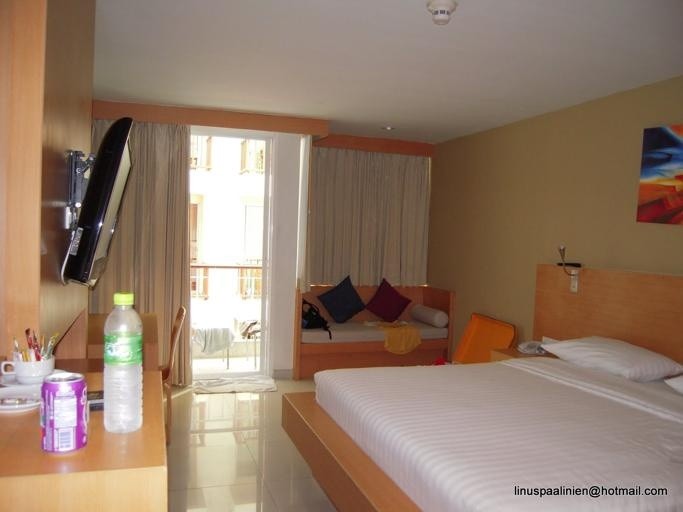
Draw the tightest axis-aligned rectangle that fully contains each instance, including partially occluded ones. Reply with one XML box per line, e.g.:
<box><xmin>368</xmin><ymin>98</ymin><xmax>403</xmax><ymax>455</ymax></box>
<box><xmin>517</xmin><ymin>341</ymin><xmax>541</xmax><ymax>354</ymax></box>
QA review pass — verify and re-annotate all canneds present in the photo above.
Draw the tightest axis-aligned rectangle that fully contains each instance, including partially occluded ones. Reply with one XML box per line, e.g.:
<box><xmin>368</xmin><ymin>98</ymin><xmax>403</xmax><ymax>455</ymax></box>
<box><xmin>40</xmin><ymin>372</ymin><xmax>90</xmax><ymax>453</ymax></box>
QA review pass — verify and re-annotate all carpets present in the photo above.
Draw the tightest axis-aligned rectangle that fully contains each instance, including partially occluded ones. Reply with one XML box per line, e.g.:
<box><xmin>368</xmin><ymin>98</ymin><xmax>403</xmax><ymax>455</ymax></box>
<box><xmin>193</xmin><ymin>376</ymin><xmax>278</xmax><ymax>395</ymax></box>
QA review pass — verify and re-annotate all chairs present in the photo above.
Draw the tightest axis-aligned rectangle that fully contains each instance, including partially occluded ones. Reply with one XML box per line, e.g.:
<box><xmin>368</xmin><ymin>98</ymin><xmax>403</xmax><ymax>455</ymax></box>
<box><xmin>162</xmin><ymin>306</ymin><xmax>186</xmax><ymax>445</ymax></box>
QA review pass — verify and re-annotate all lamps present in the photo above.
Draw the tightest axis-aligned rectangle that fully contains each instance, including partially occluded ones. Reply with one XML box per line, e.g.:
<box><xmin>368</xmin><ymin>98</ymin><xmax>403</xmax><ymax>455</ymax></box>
<box><xmin>558</xmin><ymin>244</ymin><xmax>575</xmax><ymax>277</ymax></box>
<box><xmin>427</xmin><ymin>0</ymin><xmax>456</xmax><ymax>26</ymax></box>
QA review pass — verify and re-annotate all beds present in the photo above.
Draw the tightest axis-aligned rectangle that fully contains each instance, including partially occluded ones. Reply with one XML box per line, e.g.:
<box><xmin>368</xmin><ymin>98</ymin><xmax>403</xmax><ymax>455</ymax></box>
<box><xmin>282</xmin><ymin>263</ymin><xmax>683</xmax><ymax>512</ymax></box>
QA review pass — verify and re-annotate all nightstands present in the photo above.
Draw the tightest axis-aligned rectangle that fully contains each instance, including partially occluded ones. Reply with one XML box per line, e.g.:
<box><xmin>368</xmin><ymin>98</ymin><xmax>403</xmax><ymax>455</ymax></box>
<box><xmin>490</xmin><ymin>348</ymin><xmax>555</xmax><ymax>362</ymax></box>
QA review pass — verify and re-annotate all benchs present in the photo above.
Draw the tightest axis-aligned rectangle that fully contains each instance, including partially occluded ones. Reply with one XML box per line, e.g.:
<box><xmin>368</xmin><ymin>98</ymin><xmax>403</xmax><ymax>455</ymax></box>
<box><xmin>292</xmin><ymin>285</ymin><xmax>455</xmax><ymax>381</ymax></box>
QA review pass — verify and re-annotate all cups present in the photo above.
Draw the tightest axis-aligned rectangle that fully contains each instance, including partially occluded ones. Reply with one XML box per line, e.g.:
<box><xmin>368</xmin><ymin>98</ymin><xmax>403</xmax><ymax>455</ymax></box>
<box><xmin>1</xmin><ymin>355</ymin><xmax>55</xmax><ymax>385</ymax></box>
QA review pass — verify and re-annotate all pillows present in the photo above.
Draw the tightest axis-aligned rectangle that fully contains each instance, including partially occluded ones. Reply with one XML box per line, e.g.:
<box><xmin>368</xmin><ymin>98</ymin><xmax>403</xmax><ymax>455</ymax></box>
<box><xmin>365</xmin><ymin>279</ymin><xmax>412</xmax><ymax>322</ymax></box>
<box><xmin>541</xmin><ymin>335</ymin><xmax>683</xmax><ymax>382</ymax></box>
<box><xmin>317</xmin><ymin>275</ymin><xmax>365</xmax><ymax>323</ymax></box>
<box><xmin>412</xmin><ymin>304</ymin><xmax>448</xmax><ymax>328</ymax></box>
<box><xmin>663</xmin><ymin>374</ymin><xmax>683</xmax><ymax>395</ymax></box>
<box><xmin>302</xmin><ymin>292</ymin><xmax>335</xmax><ymax>323</ymax></box>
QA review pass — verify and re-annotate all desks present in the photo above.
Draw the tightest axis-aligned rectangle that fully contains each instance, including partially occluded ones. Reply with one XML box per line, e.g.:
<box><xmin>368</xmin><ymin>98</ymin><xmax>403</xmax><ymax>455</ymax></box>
<box><xmin>0</xmin><ymin>371</ymin><xmax>169</xmax><ymax>512</ymax></box>
<box><xmin>52</xmin><ymin>307</ymin><xmax>159</xmax><ymax>372</ymax></box>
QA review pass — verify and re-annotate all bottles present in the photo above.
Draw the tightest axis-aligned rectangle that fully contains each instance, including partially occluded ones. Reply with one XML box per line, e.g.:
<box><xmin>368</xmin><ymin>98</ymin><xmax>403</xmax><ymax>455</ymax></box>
<box><xmin>103</xmin><ymin>291</ymin><xmax>144</xmax><ymax>434</ymax></box>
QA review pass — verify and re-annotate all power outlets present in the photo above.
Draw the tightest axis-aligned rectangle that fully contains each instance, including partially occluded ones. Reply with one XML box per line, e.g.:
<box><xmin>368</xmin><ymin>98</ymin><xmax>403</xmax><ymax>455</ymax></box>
<box><xmin>571</xmin><ymin>270</ymin><xmax>577</xmax><ymax>293</ymax></box>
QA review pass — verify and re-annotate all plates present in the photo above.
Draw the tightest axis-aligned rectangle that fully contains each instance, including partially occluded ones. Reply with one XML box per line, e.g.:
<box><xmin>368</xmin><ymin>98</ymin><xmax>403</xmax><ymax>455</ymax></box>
<box><xmin>0</xmin><ymin>369</ymin><xmax>68</xmax><ymax>387</ymax></box>
<box><xmin>0</xmin><ymin>386</ymin><xmax>42</xmax><ymax>413</ymax></box>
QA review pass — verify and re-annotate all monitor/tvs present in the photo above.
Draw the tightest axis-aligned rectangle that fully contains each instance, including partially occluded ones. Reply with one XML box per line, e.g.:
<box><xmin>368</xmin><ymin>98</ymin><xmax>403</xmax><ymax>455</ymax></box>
<box><xmin>66</xmin><ymin>116</ymin><xmax>135</xmax><ymax>284</ymax></box>
